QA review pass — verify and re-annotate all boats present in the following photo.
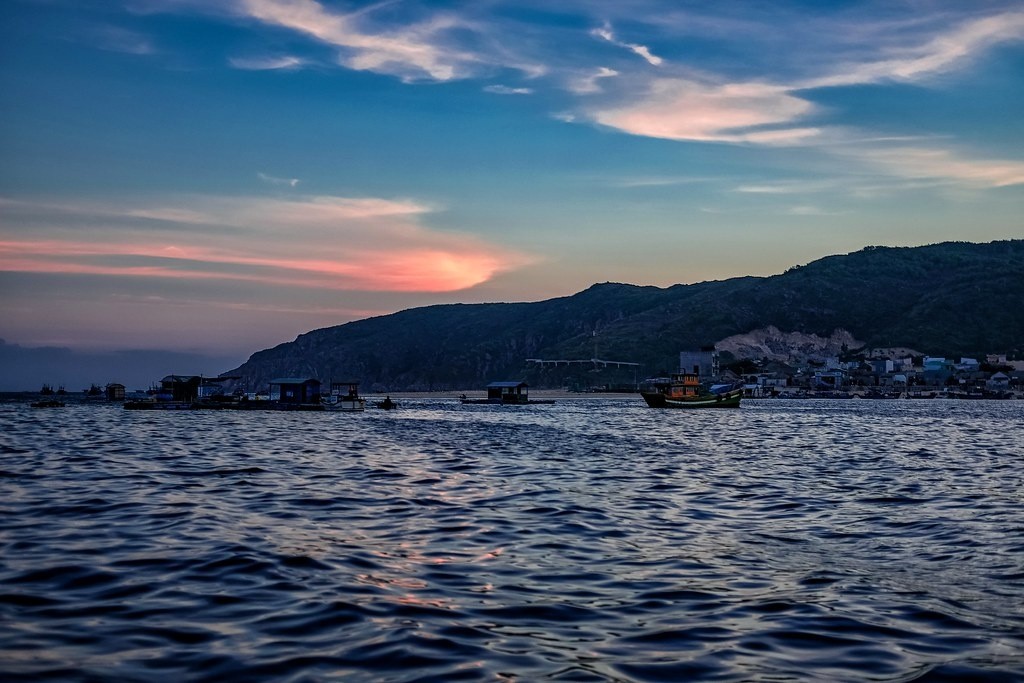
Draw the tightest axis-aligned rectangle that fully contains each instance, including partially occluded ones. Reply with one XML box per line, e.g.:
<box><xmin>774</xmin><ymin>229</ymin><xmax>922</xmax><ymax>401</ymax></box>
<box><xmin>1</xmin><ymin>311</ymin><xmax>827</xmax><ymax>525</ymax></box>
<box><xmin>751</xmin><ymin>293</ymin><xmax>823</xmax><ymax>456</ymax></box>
<box><xmin>640</xmin><ymin>387</ymin><xmax>743</xmax><ymax>408</ymax></box>
